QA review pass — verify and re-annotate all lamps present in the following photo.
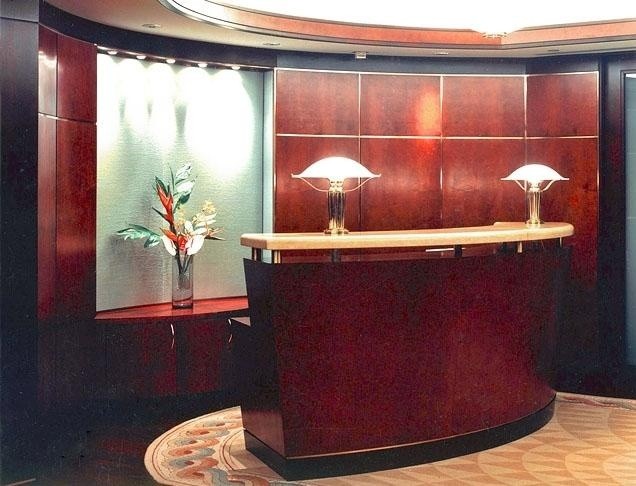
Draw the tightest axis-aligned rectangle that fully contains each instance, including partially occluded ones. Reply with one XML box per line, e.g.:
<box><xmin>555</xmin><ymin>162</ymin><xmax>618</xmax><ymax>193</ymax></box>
<box><xmin>496</xmin><ymin>162</ymin><xmax>572</xmax><ymax>227</ymax></box>
<box><xmin>290</xmin><ymin>155</ymin><xmax>383</xmax><ymax>234</ymax></box>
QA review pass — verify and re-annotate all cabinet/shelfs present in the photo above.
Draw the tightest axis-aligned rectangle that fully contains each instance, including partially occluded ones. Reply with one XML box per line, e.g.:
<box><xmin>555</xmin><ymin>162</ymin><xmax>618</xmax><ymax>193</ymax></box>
<box><xmin>92</xmin><ymin>297</ymin><xmax>250</xmax><ymax>404</ymax></box>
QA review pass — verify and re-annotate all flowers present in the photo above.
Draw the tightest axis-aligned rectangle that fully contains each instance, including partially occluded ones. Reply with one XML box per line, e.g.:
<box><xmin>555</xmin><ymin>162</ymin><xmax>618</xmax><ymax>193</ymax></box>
<box><xmin>112</xmin><ymin>162</ymin><xmax>226</xmax><ymax>285</ymax></box>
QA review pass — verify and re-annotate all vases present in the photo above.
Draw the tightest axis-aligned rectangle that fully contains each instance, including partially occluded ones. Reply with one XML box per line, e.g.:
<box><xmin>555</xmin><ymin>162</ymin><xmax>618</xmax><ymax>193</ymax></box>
<box><xmin>171</xmin><ymin>253</ymin><xmax>196</xmax><ymax>309</ymax></box>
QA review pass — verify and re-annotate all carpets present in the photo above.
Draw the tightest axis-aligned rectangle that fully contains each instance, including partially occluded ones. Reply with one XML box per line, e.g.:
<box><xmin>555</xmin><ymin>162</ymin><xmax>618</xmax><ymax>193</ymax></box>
<box><xmin>142</xmin><ymin>388</ymin><xmax>635</xmax><ymax>486</ymax></box>
